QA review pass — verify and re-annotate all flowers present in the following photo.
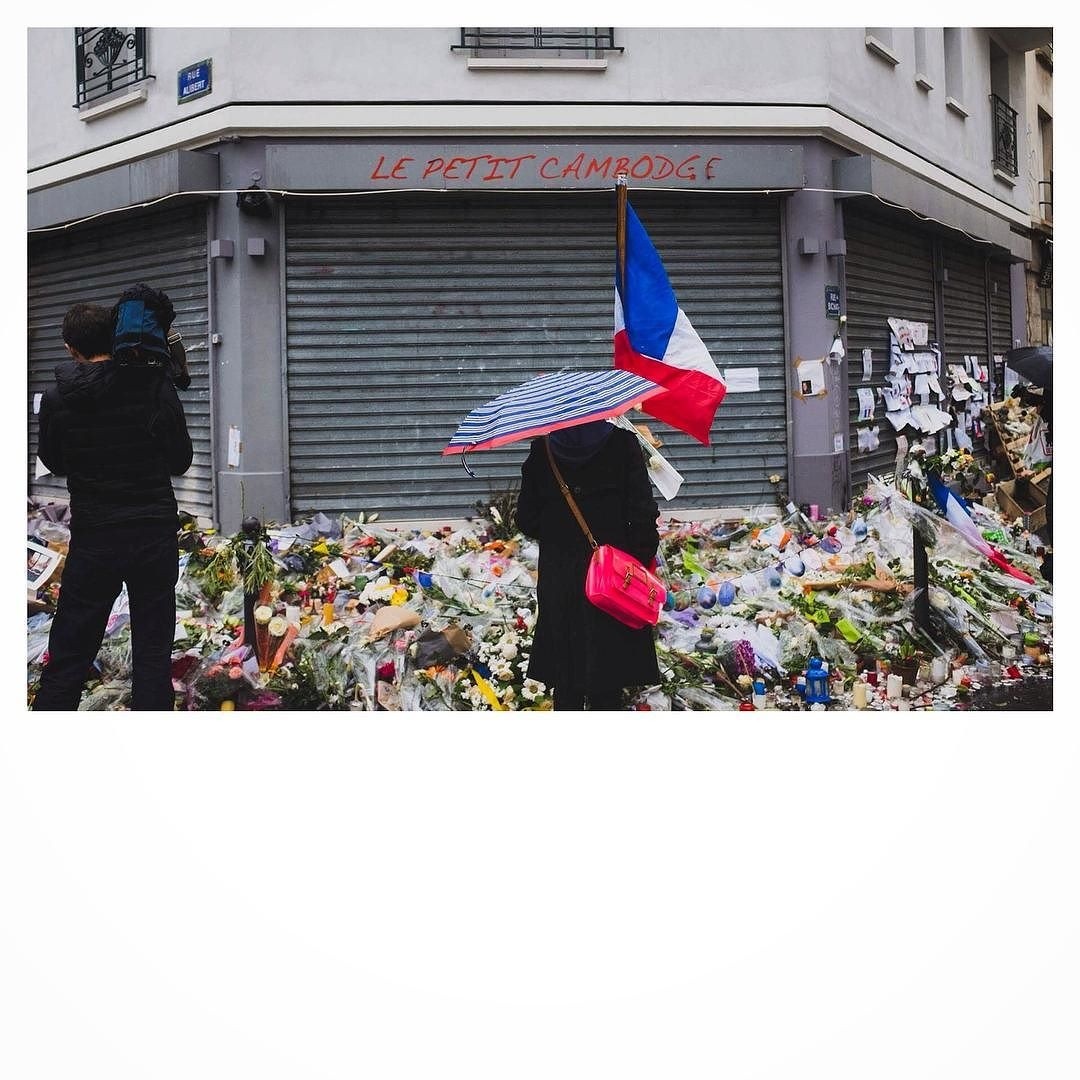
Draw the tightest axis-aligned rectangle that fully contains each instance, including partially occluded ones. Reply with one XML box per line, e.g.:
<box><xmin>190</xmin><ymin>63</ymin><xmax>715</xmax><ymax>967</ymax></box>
<box><xmin>27</xmin><ymin>444</ymin><xmax>1031</xmax><ymax>711</ymax></box>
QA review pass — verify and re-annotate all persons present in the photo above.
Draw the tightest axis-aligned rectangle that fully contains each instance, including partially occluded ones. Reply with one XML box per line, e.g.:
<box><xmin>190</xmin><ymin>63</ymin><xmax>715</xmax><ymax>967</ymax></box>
<box><xmin>32</xmin><ymin>303</ymin><xmax>194</xmax><ymax>711</ymax></box>
<box><xmin>1011</xmin><ymin>375</ymin><xmax>1052</xmax><ymax>554</ymax></box>
<box><xmin>513</xmin><ymin>418</ymin><xmax>667</xmax><ymax>711</ymax></box>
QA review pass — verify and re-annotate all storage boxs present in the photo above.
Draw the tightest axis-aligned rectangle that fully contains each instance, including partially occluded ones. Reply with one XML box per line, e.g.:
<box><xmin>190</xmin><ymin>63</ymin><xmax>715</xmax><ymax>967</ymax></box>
<box><xmin>998</xmin><ymin>478</ymin><xmax>1048</xmax><ymax>533</ymax></box>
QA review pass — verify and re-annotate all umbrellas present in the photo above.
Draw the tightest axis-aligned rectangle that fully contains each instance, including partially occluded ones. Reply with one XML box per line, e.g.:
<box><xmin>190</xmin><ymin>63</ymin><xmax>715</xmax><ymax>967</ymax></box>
<box><xmin>1006</xmin><ymin>346</ymin><xmax>1053</xmax><ymax>390</ymax></box>
<box><xmin>438</xmin><ymin>366</ymin><xmax>670</xmax><ymax>478</ymax></box>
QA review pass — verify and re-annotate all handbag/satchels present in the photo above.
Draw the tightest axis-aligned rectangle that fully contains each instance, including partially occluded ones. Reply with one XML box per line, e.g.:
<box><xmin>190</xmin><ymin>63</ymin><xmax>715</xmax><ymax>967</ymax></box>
<box><xmin>584</xmin><ymin>543</ymin><xmax>667</xmax><ymax>630</ymax></box>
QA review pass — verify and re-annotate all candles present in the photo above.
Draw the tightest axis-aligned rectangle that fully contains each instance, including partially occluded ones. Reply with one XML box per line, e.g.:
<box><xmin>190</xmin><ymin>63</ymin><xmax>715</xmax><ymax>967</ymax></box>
<box><xmin>853</xmin><ymin>682</ymin><xmax>866</xmax><ymax>707</ymax></box>
<box><xmin>867</xmin><ymin>672</ymin><xmax>878</xmax><ymax>687</ymax></box>
<box><xmin>886</xmin><ymin>673</ymin><xmax>902</xmax><ymax>699</ymax></box>
<box><xmin>953</xmin><ymin>669</ymin><xmax>963</xmax><ymax>685</ymax></box>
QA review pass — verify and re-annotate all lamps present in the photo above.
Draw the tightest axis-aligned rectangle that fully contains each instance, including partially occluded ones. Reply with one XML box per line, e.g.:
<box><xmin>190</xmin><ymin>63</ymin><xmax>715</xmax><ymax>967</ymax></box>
<box><xmin>805</xmin><ymin>658</ymin><xmax>831</xmax><ymax>703</ymax></box>
<box><xmin>695</xmin><ymin>623</ymin><xmax>718</xmax><ymax>655</ymax></box>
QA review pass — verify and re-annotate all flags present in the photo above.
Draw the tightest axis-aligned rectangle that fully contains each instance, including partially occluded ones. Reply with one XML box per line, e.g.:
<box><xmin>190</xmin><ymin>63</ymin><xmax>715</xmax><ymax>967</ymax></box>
<box><xmin>614</xmin><ymin>176</ymin><xmax>726</xmax><ymax>446</ymax></box>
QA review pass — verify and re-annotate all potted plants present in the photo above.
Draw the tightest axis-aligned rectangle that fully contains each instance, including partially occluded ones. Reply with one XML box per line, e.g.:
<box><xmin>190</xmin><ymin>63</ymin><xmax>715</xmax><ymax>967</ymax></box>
<box><xmin>893</xmin><ymin>639</ymin><xmax>921</xmax><ymax>686</ymax></box>
